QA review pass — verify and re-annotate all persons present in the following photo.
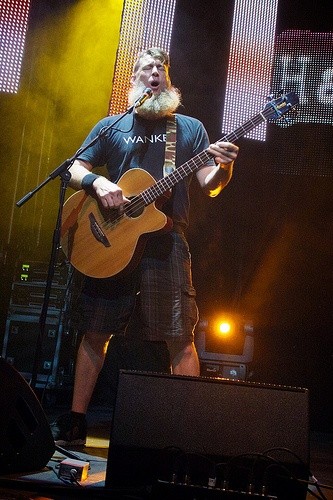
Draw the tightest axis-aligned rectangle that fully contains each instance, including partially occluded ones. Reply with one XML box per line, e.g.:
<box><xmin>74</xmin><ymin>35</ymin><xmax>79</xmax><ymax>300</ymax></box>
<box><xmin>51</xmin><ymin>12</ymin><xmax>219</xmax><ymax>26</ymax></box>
<box><xmin>52</xmin><ymin>48</ymin><xmax>239</xmax><ymax>446</ymax></box>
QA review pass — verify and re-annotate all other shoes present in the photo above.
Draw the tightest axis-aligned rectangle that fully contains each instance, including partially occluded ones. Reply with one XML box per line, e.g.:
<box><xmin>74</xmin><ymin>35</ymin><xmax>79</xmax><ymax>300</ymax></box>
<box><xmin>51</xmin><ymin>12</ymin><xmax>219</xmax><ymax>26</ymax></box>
<box><xmin>51</xmin><ymin>412</ymin><xmax>87</xmax><ymax>446</ymax></box>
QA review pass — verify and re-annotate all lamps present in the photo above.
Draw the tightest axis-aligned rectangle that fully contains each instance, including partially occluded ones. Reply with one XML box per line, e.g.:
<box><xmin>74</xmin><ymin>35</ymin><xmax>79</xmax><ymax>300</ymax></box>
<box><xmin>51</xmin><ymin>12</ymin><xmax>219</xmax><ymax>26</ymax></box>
<box><xmin>196</xmin><ymin>314</ymin><xmax>256</xmax><ymax>381</ymax></box>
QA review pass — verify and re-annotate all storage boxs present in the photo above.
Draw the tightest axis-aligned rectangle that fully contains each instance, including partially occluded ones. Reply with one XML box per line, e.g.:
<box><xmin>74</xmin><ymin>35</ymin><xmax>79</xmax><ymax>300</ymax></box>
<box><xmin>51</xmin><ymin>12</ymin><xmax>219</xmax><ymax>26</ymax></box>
<box><xmin>3</xmin><ymin>259</ymin><xmax>78</xmax><ymax>391</ymax></box>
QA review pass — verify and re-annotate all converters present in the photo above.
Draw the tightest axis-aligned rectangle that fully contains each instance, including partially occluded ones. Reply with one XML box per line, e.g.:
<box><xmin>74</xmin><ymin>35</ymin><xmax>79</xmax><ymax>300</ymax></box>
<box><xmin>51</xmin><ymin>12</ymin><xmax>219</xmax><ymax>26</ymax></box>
<box><xmin>58</xmin><ymin>457</ymin><xmax>90</xmax><ymax>482</ymax></box>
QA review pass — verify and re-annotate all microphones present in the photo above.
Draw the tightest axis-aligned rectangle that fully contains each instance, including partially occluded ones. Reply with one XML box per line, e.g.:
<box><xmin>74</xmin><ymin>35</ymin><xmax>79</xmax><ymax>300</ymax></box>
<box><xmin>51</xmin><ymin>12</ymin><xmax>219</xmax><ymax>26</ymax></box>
<box><xmin>135</xmin><ymin>88</ymin><xmax>154</xmax><ymax>108</ymax></box>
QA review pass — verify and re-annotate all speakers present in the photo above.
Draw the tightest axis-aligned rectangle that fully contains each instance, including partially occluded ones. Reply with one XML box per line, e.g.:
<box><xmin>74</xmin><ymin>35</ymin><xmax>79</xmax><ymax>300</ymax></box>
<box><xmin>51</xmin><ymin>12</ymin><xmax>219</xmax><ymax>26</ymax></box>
<box><xmin>0</xmin><ymin>355</ymin><xmax>56</xmax><ymax>475</ymax></box>
<box><xmin>105</xmin><ymin>368</ymin><xmax>310</xmax><ymax>500</ymax></box>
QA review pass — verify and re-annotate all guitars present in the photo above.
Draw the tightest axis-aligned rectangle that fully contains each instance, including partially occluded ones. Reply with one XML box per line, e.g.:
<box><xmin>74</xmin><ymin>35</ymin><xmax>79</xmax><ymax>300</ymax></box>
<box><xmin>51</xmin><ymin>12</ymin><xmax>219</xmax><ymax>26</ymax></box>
<box><xmin>61</xmin><ymin>89</ymin><xmax>300</xmax><ymax>279</ymax></box>
